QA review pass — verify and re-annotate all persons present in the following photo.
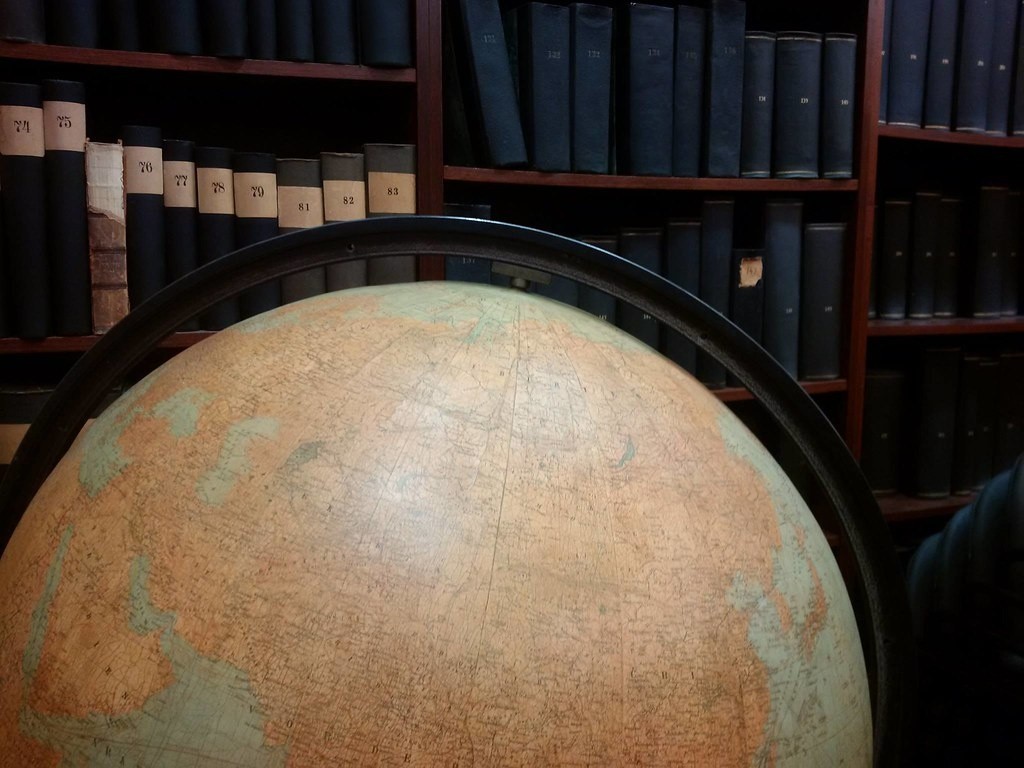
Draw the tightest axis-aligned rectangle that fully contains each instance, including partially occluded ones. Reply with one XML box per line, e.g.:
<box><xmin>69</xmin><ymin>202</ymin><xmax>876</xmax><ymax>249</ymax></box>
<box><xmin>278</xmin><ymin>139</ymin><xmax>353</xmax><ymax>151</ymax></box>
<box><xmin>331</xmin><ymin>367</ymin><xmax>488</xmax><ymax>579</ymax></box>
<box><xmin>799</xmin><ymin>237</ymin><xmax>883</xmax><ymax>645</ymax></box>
<box><xmin>905</xmin><ymin>452</ymin><xmax>1024</xmax><ymax>768</ymax></box>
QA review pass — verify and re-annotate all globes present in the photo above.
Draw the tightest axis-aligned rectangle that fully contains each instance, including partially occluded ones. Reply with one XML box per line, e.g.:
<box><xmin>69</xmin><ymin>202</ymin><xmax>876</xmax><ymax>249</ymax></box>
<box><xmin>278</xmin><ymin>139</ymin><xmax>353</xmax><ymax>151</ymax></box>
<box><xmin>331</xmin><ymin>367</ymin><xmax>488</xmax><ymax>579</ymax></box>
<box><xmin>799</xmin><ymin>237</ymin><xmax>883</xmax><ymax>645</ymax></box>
<box><xmin>1</xmin><ymin>209</ymin><xmax>908</xmax><ymax>768</ymax></box>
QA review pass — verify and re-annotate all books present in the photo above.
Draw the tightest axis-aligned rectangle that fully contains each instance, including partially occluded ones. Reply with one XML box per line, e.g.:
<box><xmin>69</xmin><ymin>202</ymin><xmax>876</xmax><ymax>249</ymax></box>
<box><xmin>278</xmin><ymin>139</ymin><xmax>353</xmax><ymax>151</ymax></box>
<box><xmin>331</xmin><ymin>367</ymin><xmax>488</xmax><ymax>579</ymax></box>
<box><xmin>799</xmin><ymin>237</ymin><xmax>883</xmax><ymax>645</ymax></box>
<box><xmin>0</xmin><ymin>0</ymin><xmax>1024</xmax><ymax>574</ymax></box>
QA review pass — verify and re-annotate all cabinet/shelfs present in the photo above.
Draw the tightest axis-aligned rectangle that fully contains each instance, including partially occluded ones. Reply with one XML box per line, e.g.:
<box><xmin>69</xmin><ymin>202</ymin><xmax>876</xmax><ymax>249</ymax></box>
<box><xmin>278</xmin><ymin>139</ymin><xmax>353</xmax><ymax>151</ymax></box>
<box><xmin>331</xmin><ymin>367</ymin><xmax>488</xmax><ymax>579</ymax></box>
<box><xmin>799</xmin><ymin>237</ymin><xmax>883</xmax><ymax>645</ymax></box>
<box><xmin>0</xmin><ymin>0</ymin><xmax>1024</xmax><ymax>600</ymax></box>
